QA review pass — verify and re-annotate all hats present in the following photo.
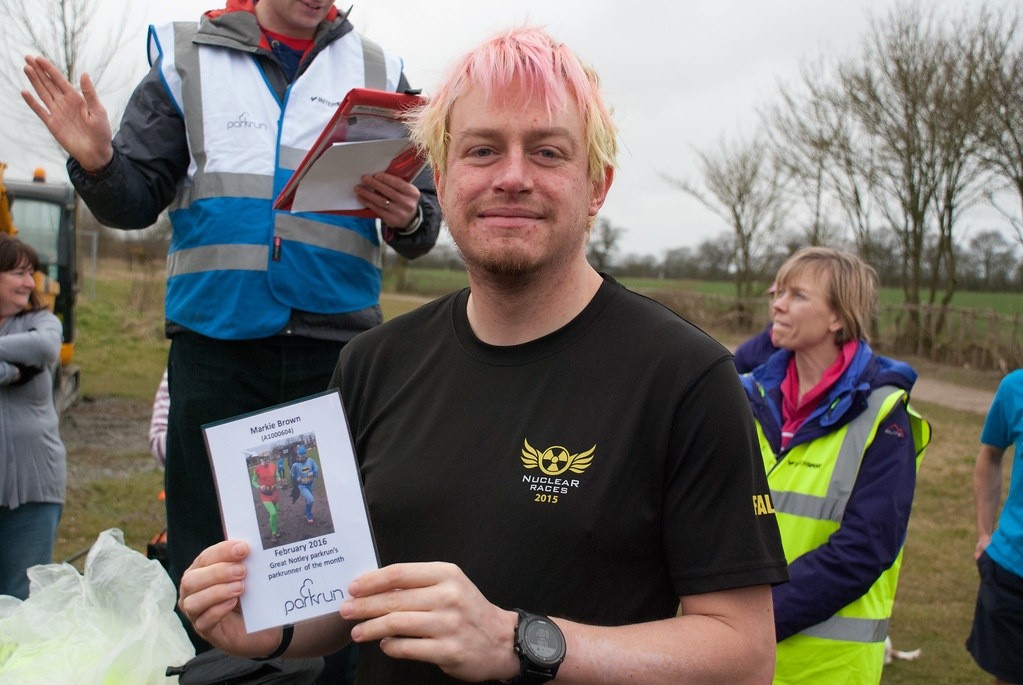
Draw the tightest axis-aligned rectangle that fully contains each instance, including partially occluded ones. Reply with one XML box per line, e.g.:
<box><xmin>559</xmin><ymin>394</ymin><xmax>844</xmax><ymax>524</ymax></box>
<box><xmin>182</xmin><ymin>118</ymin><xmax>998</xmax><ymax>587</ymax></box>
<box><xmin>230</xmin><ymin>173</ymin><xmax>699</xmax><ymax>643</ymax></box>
<box><xmin>259</xmin><ymin>450</ymin><xmax>271</xmax><ymax>457</ymax></box>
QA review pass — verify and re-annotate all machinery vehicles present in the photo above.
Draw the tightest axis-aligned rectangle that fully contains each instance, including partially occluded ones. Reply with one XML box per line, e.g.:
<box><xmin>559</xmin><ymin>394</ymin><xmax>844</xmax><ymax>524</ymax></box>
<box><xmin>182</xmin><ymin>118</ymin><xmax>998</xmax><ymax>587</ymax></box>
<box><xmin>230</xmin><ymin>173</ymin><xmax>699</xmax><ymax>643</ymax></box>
<box><xmin>0</xmin><ymin>161</ymin><xmax>82</xmax><ymax>423</ymax></box>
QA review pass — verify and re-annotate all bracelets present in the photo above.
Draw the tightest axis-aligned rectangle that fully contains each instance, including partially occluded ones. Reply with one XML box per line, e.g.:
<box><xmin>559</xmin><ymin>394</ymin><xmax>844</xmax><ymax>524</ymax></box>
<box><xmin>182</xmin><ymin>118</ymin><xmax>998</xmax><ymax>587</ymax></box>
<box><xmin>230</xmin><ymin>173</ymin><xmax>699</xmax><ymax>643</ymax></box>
<box><xmin>249</xmin><ymin>624</ymin><xmax>294</xmax><ymax>662</ymax></box>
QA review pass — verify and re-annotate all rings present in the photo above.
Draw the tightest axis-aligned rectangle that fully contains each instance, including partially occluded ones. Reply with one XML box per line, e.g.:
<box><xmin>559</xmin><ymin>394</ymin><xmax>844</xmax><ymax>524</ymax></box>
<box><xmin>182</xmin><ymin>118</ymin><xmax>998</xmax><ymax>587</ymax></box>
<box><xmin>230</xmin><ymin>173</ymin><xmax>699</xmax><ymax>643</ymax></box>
<box><xmin>386</xmin><ymin>200</ymin><xmax>390</xmax><ymax>210</ymax></box>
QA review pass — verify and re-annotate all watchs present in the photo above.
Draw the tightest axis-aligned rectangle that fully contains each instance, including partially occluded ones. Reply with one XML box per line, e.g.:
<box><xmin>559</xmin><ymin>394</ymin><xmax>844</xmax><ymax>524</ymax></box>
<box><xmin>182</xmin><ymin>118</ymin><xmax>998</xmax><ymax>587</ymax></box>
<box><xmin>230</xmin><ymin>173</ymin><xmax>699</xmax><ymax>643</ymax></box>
<box><xmin>513</xmin><ymin>608</ymin><xmax>567</xmax><ymax>685</ymax></box>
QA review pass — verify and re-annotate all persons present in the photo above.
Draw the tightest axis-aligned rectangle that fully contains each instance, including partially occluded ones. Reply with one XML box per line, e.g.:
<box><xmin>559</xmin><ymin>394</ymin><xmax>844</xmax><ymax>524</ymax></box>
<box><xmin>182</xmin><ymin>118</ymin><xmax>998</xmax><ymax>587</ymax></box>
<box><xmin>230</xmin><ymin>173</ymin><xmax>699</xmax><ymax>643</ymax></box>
<box><xmin>177</xmin><ymin>28</ymin><xmax>791</xmax><ymax>684</ymax></box>
<box><xmin>148</xmin><ymin>366</ymin><xmax>170</xmax><ymax>464</ymax></box>
<box><xmin>251</xmin><ymin>445</ymin><xmax>319</xmax><ymax>539</ymax></box>
<box><xmin>21</xmin><ymin>1</ymin><xmax>442</xmax><ymax>657</ymax></box>
<box><xmin>0</xmin><ymin>235</ymin><xmax>66</xmax><ymax>601</ymax></box>
<box><xmin>732</xmin><ymin>246</ymin><xmax>1023</xmax><ymax>685</ymax></box>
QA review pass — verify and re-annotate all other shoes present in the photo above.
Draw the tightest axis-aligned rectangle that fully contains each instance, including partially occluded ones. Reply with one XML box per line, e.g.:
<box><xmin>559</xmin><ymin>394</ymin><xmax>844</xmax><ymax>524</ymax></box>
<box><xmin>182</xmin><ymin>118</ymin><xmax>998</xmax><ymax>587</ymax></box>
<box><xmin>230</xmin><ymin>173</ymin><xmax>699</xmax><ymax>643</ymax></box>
<box><xmin>307</xmin><ymin>519</ymin><xmax>313</xmax><ymax>523</ymax></box>
<box><xmin>276</xmin><ymin>534</ymin><xmax>280</xmax><ymax>537</ymax></box>
<box><xmin>272</xmin><ymin>536</ymin><xmax>276</xmax><ymax>541</ymax></box>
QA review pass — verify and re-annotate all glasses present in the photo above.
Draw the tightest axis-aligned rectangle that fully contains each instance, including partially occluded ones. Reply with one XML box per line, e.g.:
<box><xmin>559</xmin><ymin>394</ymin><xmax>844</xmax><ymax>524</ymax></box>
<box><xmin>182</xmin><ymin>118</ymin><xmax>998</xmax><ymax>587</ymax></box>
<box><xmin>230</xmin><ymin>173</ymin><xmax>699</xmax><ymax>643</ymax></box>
<box><xmin>301</xmin><ymin>451</ymin><xmax>306</xmax><ymax>454</ymax></box>
